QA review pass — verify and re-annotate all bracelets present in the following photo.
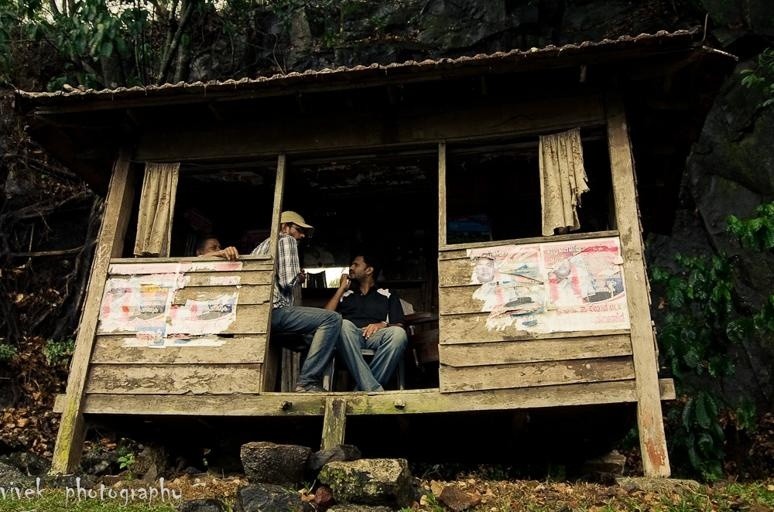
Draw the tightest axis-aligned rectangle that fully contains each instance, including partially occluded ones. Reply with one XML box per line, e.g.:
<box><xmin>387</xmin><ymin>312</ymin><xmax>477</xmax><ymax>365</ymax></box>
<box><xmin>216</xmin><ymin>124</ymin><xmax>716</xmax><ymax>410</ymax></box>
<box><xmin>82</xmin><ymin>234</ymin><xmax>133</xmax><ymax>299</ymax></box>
<box><xmin>380</xmin><ymin>320</ymin><xmax>389</xmax><ymax>327</ymax></box>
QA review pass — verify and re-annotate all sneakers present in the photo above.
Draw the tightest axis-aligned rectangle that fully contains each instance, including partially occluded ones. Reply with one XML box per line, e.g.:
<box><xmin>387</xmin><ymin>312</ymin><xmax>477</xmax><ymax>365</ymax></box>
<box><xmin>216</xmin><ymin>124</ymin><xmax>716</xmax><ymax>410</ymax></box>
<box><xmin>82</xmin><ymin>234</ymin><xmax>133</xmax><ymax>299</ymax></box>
<box><xmin>296</xmin><ymin>384</ymin><xmax>327</xmax><ymax>391</ymax></box>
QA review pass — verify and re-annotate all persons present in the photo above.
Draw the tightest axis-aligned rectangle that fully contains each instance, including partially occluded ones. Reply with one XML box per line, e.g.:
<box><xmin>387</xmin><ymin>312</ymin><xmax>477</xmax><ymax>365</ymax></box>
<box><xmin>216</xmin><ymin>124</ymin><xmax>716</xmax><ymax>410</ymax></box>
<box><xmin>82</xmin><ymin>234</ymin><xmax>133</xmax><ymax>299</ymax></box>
<box><xmin>248</xmin><ymin>211</ymin><xmax>343</xmax><ymax>391</ymax></box>
<box><xmin>195</xmin><ymin>235</ymin><xmax>237</xmax><ymax>261</ymax></box>
<box><xmin>475</xmin><ymin>256</ymin><xmax>496</xmax><ymax>284</ymax></box>
<box><xmin>324</xmin><ymin>248</ymin><xmax>411</xmax><ymax>393</ymax></box>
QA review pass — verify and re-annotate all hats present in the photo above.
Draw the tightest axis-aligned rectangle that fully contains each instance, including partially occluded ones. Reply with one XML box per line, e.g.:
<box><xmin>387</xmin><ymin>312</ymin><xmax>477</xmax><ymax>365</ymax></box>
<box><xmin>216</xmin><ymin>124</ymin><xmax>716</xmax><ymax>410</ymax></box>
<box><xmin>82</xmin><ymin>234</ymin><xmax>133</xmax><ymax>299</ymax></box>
<box><xmin>280</xmin><ymin>211</ymin><xmax>313</xmax><ymax>228</ymax></box>
